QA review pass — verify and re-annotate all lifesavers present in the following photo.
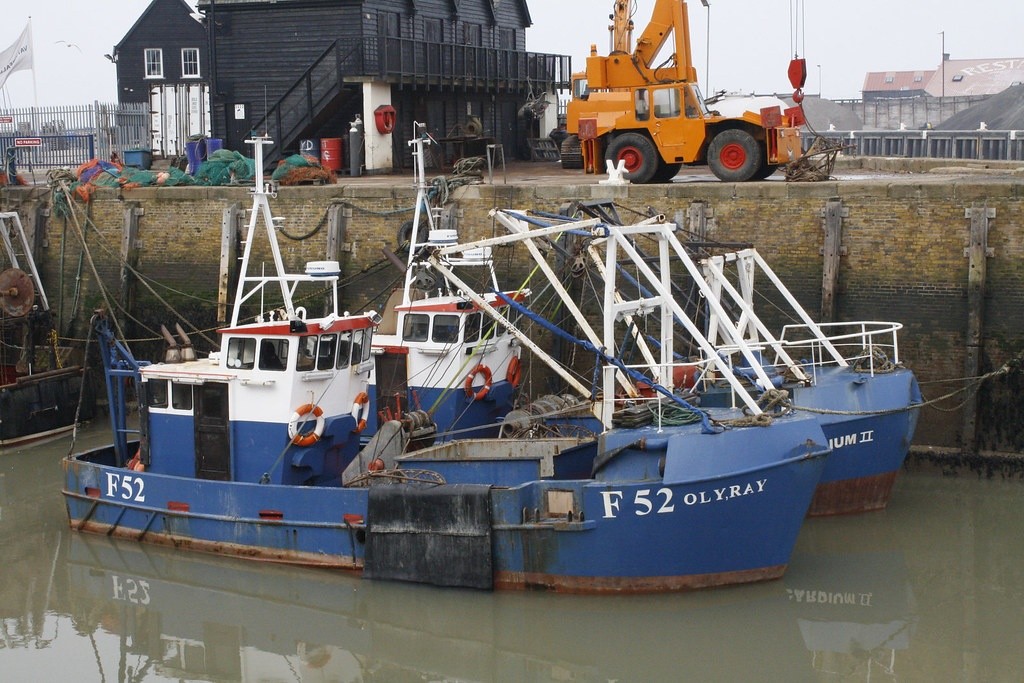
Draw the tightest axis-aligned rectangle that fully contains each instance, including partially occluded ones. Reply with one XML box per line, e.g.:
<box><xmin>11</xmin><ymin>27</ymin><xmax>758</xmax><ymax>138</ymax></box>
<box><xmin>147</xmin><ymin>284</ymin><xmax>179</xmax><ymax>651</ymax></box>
<box><xmin>507</xmin><ymin>360</ymin><xmax>522</xmax><ymax>386</ymax></box>
<box><xmin>355</xmin><ymin>393</ymin><xmax>370</xmax><ymax>432</ymax></box>
<box><xmin>466</xmin><ymin>368</ymin><xmax>492</xmax><ymax>398</ymax></box>
<box><xmin>289</xmin><ymin>403</ymin><xmax>326</xmax><ymax>446</ymax></box>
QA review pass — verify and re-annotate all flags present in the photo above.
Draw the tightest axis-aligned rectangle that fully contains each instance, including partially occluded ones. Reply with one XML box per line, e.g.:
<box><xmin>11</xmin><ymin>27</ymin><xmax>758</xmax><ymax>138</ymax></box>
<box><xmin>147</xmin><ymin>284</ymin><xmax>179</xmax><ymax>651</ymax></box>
<box><xmin>0</xmin><ymin>22</ymin><xmax>33</xmax><ymax>90</ymax></box>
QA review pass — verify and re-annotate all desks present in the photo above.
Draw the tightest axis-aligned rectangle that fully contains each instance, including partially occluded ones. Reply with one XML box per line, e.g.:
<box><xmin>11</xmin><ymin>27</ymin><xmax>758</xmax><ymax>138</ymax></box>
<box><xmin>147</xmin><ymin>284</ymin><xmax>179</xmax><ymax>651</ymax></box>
<box><xmin>425</xmin><ymin>135</ymin><xmax>497</xmax><ymax>173</ymax></box>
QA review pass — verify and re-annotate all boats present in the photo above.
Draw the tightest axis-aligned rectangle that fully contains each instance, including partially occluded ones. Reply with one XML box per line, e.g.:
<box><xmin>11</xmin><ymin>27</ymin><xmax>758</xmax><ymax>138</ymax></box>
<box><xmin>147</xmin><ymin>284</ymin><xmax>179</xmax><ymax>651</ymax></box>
<box><xmin>0</xmin><ymin>116</ymin><xmax>927</xmax><ymax>593</ymax></box>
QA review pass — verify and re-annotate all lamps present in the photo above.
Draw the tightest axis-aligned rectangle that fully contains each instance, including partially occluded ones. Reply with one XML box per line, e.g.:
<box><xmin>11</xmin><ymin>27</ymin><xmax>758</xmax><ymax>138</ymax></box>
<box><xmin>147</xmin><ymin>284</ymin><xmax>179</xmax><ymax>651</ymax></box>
<box><xmin>289</xmin><ymin>318</ymin><xmax>308</xmax><ymax>335</ymax></box>
<box><xmin>365</xmin><ymin>310</ymin><xmax>382</xmax><ymax>326</ymax></box>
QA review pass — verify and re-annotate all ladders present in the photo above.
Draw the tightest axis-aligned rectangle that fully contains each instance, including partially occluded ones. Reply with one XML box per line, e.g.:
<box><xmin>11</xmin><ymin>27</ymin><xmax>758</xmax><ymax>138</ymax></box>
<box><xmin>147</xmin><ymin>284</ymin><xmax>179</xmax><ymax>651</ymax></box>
<box><xmin>0</xmin><ymin>211</ymin><xmax>51</xmax><ymax>312</ymax></box>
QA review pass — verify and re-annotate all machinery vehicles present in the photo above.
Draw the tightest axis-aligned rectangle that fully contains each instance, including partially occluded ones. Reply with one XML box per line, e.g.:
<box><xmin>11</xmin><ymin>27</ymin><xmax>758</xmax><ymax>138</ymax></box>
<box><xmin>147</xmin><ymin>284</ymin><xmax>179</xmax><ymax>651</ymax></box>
<box><xmin>565</xmin><ymin>0</ymin><xmax>854</xmax><ymax>184</ymax></box>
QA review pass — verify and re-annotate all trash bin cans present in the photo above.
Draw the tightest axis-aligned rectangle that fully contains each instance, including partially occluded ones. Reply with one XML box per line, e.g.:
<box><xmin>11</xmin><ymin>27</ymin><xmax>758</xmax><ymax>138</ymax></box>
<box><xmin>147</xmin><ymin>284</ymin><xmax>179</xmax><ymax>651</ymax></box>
<box><xmin>122</xmin><ymin>150</ymin><xmax>153</xmax><ymax>169</ymax></box>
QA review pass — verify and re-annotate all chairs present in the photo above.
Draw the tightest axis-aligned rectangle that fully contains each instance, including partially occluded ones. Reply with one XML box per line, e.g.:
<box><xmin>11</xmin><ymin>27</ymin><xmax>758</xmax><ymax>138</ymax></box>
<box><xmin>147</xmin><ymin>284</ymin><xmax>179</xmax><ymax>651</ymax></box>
<box><xmin>636</xmin><ymin>100</ymin><xmax>649</xmax><ymax>121</ymax></box>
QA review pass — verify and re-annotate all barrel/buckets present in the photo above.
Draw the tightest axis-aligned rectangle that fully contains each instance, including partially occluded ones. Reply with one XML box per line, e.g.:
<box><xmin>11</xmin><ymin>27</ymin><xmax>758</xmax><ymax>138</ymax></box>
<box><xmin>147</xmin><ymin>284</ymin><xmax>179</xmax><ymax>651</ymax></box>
<box><xmin>300</xmin><ymin>137</ymin><xmax>320</xmax><ymax>162</ymax></box>
<box><xmin>320</xmin><ymin>138</ymin><xmax>343</xmax><ymax>177</ymax></box>
